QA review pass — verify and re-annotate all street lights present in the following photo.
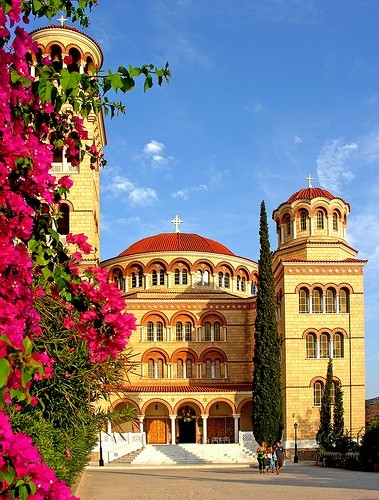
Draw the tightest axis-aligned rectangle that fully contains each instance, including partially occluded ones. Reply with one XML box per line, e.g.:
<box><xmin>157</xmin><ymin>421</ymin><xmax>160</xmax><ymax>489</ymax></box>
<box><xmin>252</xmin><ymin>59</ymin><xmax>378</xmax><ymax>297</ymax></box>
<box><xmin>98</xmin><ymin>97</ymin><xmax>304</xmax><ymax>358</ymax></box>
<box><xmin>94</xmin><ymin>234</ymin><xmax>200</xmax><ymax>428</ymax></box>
<box><xmin>293</xmin><ymin>423</ymin><xmax>299</xmax><ymax>463</ymax></box>
<box><xmin>97</xmin><ymin>422</ymin><xmax>104</xmax><ymax>466</ymax></box>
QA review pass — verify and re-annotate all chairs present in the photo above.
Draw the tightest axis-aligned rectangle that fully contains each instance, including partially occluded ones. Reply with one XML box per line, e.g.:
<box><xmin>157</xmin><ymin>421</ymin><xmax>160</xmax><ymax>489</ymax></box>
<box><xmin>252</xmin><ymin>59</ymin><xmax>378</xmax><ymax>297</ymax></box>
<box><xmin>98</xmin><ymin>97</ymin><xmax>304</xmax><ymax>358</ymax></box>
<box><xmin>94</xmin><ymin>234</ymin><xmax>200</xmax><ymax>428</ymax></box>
<box><xmin>218</xmin><ymin>437</ymin><xmax>225</xmax><ymax>444</ymax></box>
<box><xmin>224</xmin><ymin>437</ymin><xmax>230</xmax><ymax>443</ymax></box>
<box><xmin>202</xmin><ymin>437</ymin><xmax>209</xmax><ymax>443</ymax></box>
<box><xmin>212</xmin><ymin>437</ymin><xmax>219</xmax><ymax>444</ymax></box>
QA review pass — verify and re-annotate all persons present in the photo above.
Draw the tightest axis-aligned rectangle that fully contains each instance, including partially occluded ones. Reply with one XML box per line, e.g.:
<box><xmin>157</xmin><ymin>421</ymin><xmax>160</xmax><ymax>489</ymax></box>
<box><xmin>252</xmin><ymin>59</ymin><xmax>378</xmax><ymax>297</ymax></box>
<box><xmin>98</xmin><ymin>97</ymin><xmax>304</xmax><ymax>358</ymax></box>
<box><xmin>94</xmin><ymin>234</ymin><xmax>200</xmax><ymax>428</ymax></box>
<box><xmin>256</xmin><ymin>441</ymin><xmax>285</xmax><ymax>476</ymax></box>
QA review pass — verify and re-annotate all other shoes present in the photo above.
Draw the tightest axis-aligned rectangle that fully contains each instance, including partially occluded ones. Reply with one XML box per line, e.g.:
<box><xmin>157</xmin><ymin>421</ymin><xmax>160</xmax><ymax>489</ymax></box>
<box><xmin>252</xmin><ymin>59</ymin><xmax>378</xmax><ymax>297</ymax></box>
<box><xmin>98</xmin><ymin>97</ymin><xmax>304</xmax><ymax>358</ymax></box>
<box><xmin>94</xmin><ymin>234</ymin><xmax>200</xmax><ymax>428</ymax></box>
<box><xmin>259</xmin><ymin>468</ymin><xmax>281</xmax><ymax>475</ymax></box>
